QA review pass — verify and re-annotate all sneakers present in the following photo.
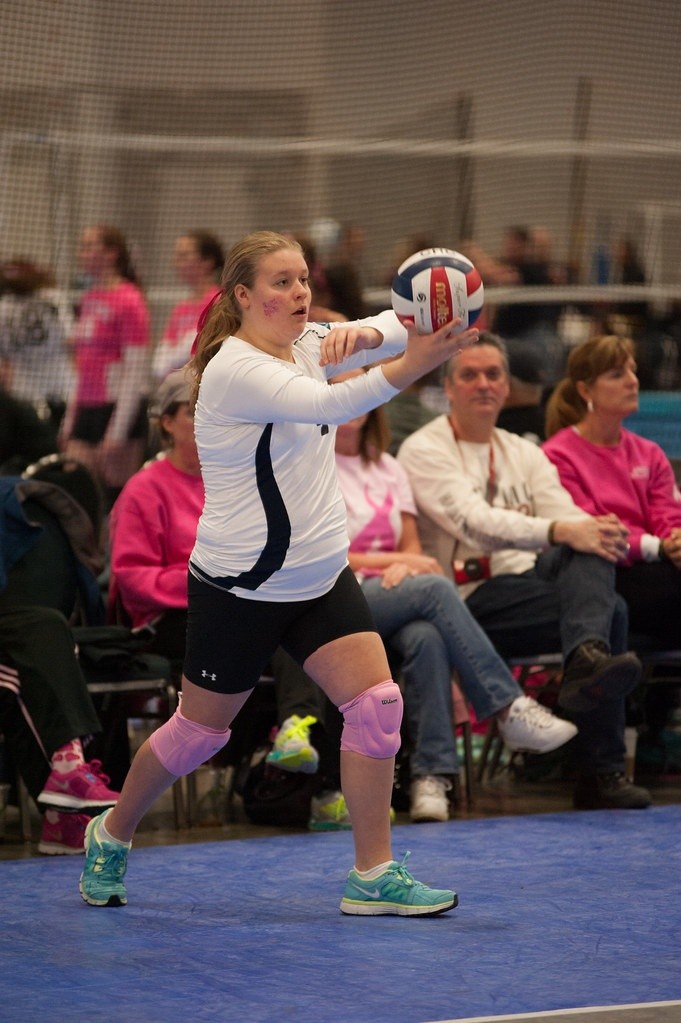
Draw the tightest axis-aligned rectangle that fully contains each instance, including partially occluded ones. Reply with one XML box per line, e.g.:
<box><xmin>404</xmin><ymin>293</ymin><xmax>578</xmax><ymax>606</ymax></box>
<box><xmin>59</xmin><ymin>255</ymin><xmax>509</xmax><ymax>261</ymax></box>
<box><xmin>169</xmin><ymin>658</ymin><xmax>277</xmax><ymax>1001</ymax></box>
<box><xmin>574</xmin><ymin>768</ymin><xmax>652</xmax><ymax>810</ymax></box>
<box><xmin>309</xmin><ymin>791</ymin><xmax>396</xmax><ymax>830</ymax></box>
<box><xmin>409</xmin><ymin>775</ymin><xmax>452</xmax><ymax>821</ymax></box>
<box><xmin>496</xmin><ymin>695</ymin><xmax>578</xmax><ymax>754</ymax></box>
<box><xmin>79</xmin><ymin>807</ymin><xmax>131</xmax><ymax>906</ymax></box>
<box><xmin>340</xmin><ymin>851</ymin><xmax>458</xmax><ymax>917</ymax></box>
<box><xmin>38</xmin><ymin>807</ymin><xmax>93</xmax><ymax>855</ymax></box>
<box><xmin>558</xmin><ymin>641</ymin><xmax>642</xmax><ymax>711</ymax></box>
<box><xmin>265</xmin><ymin>714</ymin><xmax>319</xmax><ymax>774</ymax></box>
<box><xmin>37</xmin><ymin>759</ymin><xmax>120</xmax><ymax>815</ymax></box>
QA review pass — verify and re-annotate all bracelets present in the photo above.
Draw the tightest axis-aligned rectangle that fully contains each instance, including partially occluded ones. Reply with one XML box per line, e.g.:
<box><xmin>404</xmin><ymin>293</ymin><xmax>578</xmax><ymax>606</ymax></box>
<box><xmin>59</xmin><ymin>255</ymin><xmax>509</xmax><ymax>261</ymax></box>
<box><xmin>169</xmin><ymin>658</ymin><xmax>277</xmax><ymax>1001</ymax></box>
<box><xmin>547</xmin><ymin>521</ymin><xmax>560</xmax><ymax>547</ymax></box>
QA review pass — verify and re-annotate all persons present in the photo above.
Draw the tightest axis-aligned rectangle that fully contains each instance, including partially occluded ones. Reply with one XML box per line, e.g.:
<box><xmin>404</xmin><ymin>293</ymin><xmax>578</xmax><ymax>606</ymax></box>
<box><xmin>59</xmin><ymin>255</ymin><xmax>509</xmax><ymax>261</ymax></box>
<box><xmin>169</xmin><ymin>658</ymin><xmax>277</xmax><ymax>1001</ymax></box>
<box><xmin>333</xmin><ymin>361</ymin><xmax>578</xmax><ymax>822</ymax></box>
<box><xmin>147</xmin><ymin>233</ymin><xmax>225</xmax><ymax>389</ymax></box>
<box><xmin>61</xmin><ymin>232</ymin><xmax>156</xmax><ymax>513</ymax></box>
<box><xmin>0</xmin><ymin>254</ymin><xmax>80</xmax><ymax>483</ymax></box>
<box><xmin>78</xmin><ymin>228</ymin><xmax>479</xmax><ymax>916</ymax></box>
<box><xmin>397</xmin><ymin>336</ymin><xmax>651</xmax><ymax>811</ymax></box>
<box><xmin>0</xmin><ymin>473</ymin><xmax>122</xmax><ymax>859</ymax></box>
<box><xmin>283</xmin><ymin>226</ymin><xmax>369</xmax><ymax>325</ymax></box>
<box><xmin>535</xmin><ymin>334</ymin><xmax>681</xmax><ymax>798</ymax></box>
<box><xmin>388</xmin><ymin>219</ymin><xmax>566</xmax><ymax>338</ymax></box>
<box><xmin>609</xmin><ymin>234</ymin><xmax>655</xmax><ymax>334</ymax></box>
<box><xmin>107</xmin><ymin>367</ymin><xmax>397</xmax><ymax>832</ymax></box>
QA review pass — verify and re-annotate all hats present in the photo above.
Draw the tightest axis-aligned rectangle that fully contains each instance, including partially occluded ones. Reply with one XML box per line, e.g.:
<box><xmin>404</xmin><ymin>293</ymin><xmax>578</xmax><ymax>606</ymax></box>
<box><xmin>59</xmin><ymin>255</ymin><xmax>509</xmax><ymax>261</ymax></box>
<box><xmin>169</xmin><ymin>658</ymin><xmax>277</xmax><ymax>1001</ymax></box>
<box><xmin>157</xmin><ymin>367</ymin><xmax>199</xmax><ymax>415</ymax></box>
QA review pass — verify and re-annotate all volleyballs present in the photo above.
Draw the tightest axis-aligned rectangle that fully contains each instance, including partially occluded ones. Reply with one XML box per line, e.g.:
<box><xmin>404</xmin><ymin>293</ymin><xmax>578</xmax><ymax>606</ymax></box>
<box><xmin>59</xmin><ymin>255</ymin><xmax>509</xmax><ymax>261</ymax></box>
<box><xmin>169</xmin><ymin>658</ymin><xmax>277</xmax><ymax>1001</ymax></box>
<box><xmin>391</xmin><ymin>248</ymin><xmax>486</xmax><ymax>338</ymax></box>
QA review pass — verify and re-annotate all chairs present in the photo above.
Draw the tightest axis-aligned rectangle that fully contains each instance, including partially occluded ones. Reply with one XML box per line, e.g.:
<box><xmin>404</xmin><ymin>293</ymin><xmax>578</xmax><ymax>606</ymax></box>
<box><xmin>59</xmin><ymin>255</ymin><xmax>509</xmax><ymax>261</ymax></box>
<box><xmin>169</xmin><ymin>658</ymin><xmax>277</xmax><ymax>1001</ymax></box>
<box><xmin>0</xmin><ymin>456</ymin><xmax>681</xmax><ymax>829</ymax></box>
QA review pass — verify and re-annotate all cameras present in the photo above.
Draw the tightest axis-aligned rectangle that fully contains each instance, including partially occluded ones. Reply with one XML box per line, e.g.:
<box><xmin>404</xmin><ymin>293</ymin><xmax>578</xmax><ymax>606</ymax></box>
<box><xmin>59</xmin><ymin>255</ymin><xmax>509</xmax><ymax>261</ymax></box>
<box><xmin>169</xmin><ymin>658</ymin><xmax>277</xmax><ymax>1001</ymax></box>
<box><xmin>451</xmin><ymin>556</ymin><xmax>496</xmax><ymax>585</ymax></box>
